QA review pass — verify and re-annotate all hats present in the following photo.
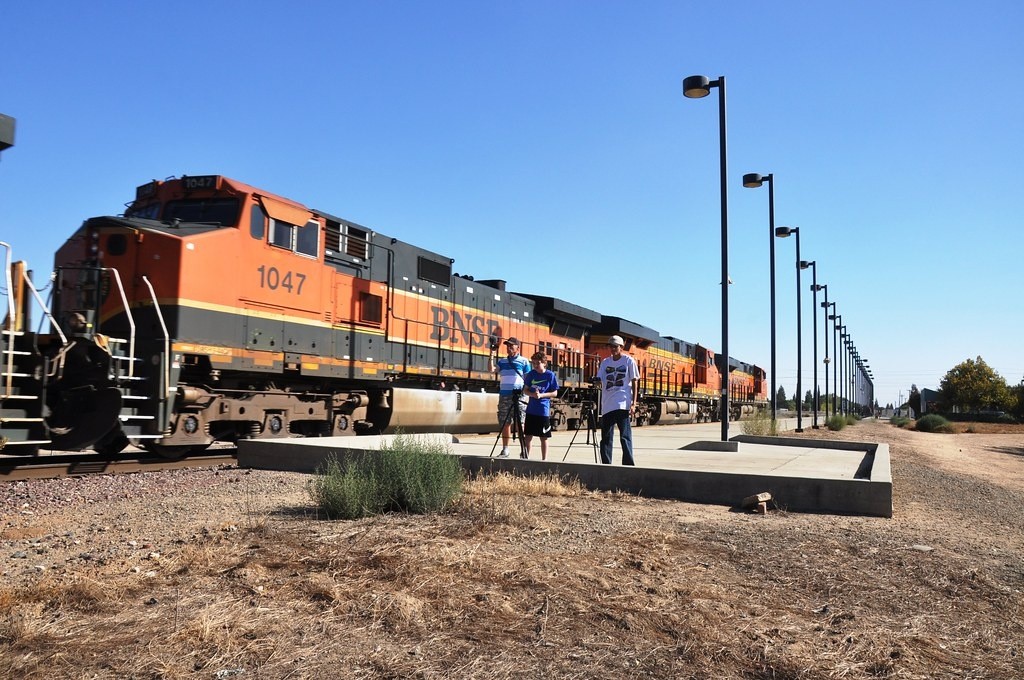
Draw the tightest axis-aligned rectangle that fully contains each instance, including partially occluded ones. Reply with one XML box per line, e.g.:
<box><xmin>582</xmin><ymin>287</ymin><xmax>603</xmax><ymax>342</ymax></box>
<box><xmin>604</xmin><ymin>334</ymin><xmax>625</xmax><ymax>347</ymax></box>
<box><xmin>503</xmin><ymin>337</ymin><xmax>519</xmax><ymax>346</ymax></box>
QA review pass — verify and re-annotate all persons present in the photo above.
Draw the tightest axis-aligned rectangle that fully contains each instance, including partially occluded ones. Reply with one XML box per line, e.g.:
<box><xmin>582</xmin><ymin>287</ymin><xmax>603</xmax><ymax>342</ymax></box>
<box><xmin>595</xmin><ymin>335</ymin><xmax>641</xmax><ymax>466</ymax></box>
<box><xmin>488</xmin><ymin>337</ymin><xmax>530</xmax><ymax>458</ymax></box>
<box><xmin>522</xmin><ymin>351</ymin><xmax>559</xmax><ymax>461</ymax></box>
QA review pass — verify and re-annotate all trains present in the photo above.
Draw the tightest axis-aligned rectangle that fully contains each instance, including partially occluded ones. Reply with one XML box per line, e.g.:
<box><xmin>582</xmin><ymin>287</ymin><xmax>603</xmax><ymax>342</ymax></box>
<box><xmin>0</xmin><ymin>113</ymin><xmax>766</xmax><ymax>470</ymax></box>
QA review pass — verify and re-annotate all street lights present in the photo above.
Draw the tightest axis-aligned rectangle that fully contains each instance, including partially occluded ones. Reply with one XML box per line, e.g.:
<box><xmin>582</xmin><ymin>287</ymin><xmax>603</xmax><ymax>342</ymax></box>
<box><xmin>810</xmin><ymin>284</ymin><xmax>829</xmax><ymax>427</ymax></box>
<box><xmin>796</xmin><ymin>260</ymin><xmax>820</xmax><ymax>429</ymax></box>
<box><xmin>821</xmin><ymin>300</ymin><xmax>876</xmax><ymax>423</ymax></box>
<box><xmin>681</xmin><ymin>76</ymin><xmax>730</xmax><ymax>442</ymax></box>
<box><xmin>775</xmin><ymin>226</ymin><xmax>803</xmax><ymax>433</ymax></box>
<box><xmin>742</xmin><ymin>172</ymin><xmax>779</xmax><ymax>437</ymax></box>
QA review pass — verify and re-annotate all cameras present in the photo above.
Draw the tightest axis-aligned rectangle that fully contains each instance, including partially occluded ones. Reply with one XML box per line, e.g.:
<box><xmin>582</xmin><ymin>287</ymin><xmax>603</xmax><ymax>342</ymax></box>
<box><xmin>491</xmin><ymin>344</ymin><xmax>499</xmax><ymax>349</ymax></box>
<box><xmin>587</xmin><ymin>376</ymin><xmax>601</xmax><ymax>383</ymax></box>
<box><xmin>511</xmin><ymin>388</ymin><xmax>521</xmax><ymax>395</ymax></box>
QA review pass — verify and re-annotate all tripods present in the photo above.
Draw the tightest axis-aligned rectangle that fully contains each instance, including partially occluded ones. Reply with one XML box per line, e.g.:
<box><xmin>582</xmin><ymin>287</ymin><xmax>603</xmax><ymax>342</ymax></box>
<box><xmin>562</xmin><ymin>385</ymin><xmax>601</xmax><ymax>464</ymax></box>
<box><xmin>489</xmin><ymin>395</ymin><xmax>528</xmax><ymax>459</ymax></box>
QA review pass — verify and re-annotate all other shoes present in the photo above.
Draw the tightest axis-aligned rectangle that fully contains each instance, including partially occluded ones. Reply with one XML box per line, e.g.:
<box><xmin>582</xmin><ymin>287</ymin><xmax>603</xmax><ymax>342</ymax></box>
<box><xmin>497</xmin><ymin>451</ymin><xmax>510</xmax><ymax>458</ymax></box>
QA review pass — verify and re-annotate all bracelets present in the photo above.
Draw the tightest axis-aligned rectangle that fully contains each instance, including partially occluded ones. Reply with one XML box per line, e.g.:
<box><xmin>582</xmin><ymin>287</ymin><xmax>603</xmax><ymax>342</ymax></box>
<box><xmin>489</xmin><ymin>359</ymin><xmax>493</xmax><ymax>360</ymax></box>
